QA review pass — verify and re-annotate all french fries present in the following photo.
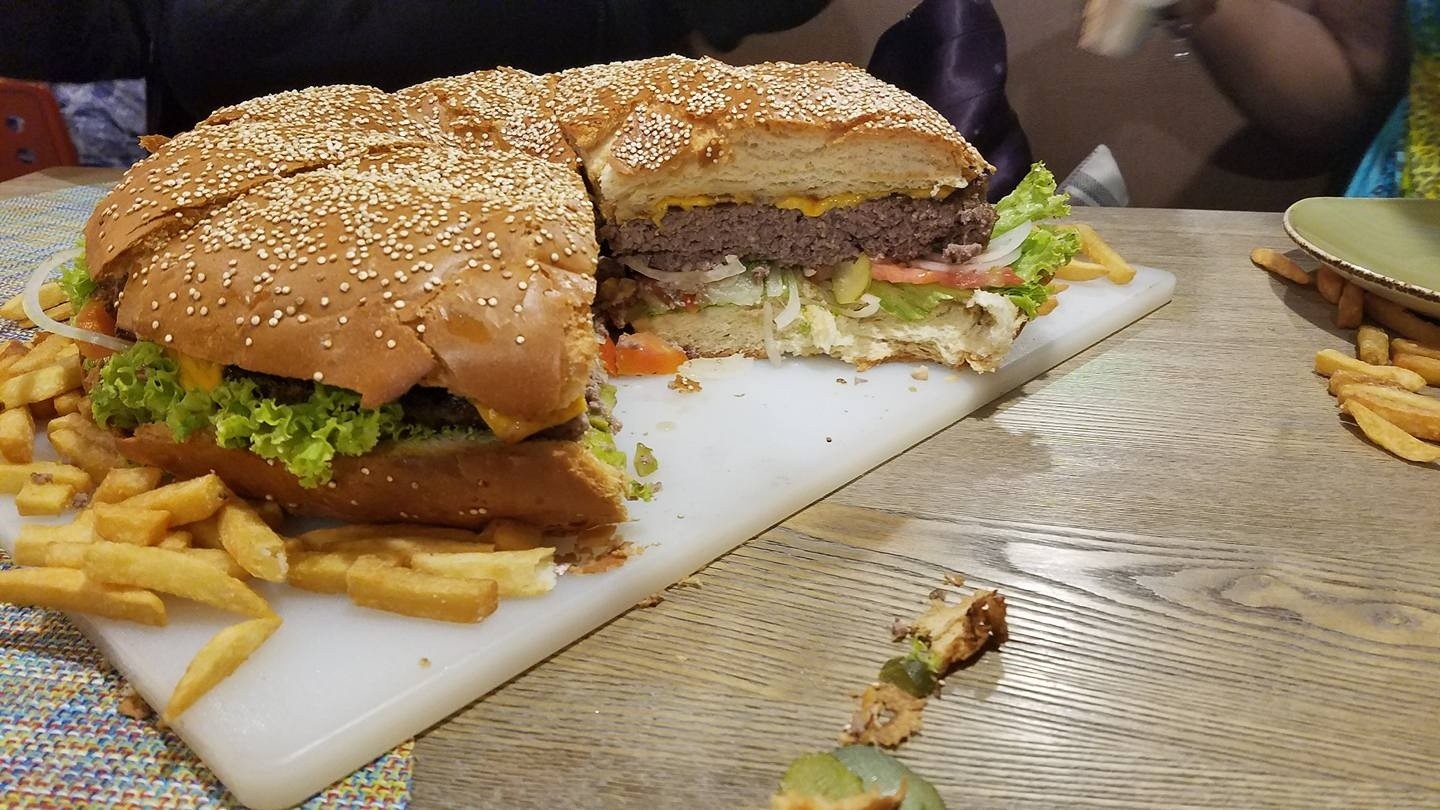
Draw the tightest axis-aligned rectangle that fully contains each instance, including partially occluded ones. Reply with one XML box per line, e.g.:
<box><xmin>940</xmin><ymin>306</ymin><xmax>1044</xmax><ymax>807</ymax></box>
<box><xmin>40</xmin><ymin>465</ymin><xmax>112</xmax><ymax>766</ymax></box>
<box><xmin>1251</xmin><ymin>246</ymin><xmax>1439</xmax><ymax>464</ymax></box>
<box><xmin>1056</xmin><ymin>223</ymin><xmax>1135</xmax><ymax>284</ymax></box>
<box><xmin>3</xmin><ymin>284</ymin><xmax>558</xmax><ymax>726</ymax></box>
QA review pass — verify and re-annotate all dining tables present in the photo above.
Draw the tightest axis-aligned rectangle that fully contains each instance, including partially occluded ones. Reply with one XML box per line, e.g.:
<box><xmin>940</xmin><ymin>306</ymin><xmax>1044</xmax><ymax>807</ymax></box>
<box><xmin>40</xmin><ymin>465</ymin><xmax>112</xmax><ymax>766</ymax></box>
<box><xmin>1</xmin><ymin>163</ymin><xmax>1439</xmax><ymax>805</ymax></box>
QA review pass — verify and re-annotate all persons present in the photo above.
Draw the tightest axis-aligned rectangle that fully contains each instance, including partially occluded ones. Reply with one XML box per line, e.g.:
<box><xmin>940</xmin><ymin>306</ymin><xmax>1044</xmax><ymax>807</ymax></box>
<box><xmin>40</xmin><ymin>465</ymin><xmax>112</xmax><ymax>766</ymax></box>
<box><xmin>0</xmin><ymin>1</ymin><xmax>855</xmax><ymax>187</ymax></box>
<box><xmin>1074</xmin><ymin>1</ymin><xmax>1440</xmax><ymax>197</ymax></box>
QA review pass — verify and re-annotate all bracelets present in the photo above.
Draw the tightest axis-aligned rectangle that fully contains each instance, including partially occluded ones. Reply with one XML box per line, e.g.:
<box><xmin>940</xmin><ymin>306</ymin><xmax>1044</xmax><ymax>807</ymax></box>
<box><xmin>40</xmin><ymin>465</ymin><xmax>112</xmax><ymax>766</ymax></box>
<box><xmin>1141</xmin><ymin>1</ymin><xmax>1220</xmax><ymax>61</ymax></box>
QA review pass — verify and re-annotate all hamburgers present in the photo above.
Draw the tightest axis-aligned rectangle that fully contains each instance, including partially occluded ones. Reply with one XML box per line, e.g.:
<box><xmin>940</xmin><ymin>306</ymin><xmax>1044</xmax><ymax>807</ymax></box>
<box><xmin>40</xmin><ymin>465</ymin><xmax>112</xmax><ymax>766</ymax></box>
<box><xmin>68</xmin><ymin>51</ymin><xmax>1086</xmax><ymax>559</ymax></box>
